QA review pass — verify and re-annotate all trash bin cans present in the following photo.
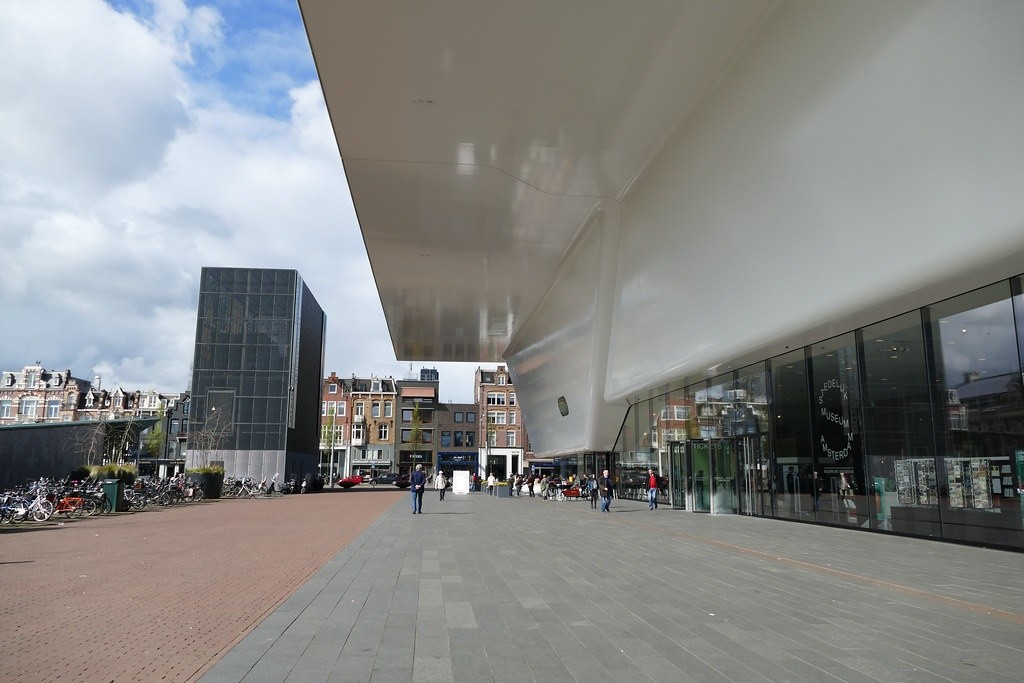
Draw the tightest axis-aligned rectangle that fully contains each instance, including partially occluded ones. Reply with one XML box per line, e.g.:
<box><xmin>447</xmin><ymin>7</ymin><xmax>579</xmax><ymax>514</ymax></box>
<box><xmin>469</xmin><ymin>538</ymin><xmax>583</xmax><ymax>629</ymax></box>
<box><xmin>101</xmin><ymin>477</ymin><xmax>125</xmax><ymax>513</ymax></box>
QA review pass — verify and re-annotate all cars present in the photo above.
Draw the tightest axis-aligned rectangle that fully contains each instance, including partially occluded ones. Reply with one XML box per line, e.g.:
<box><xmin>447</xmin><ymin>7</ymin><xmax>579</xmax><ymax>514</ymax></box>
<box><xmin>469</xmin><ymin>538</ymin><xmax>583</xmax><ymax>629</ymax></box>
<box><xmin>368</xmin><ymin>473</ymin><xmax>399</xmax><ymax>485</ymax></box>
<box><xmin>392</xmin><ymin>472</ymin><xmax>427</xmax><ymax>488</ymax></box>
<box><xmin>338</xmin><ymin>475</ymin><xmax>364</xmax><ymax>486</ymax></box>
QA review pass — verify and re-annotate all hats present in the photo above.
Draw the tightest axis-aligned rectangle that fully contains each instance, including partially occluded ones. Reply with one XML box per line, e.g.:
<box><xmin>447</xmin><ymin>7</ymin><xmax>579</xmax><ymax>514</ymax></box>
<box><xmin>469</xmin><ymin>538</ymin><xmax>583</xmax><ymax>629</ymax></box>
<box><xmin>439</xmin><ymin>471</ymin><xmax>443</xmax><ymax>473</ymax></box>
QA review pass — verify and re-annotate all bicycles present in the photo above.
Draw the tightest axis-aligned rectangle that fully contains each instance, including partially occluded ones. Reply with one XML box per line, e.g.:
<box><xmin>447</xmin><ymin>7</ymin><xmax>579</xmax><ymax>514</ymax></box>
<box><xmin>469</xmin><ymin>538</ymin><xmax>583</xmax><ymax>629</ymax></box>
<box><xmin>0</xmin><ymin>470</ymin><xmax>309</xmax><ymax>524</ymax></box>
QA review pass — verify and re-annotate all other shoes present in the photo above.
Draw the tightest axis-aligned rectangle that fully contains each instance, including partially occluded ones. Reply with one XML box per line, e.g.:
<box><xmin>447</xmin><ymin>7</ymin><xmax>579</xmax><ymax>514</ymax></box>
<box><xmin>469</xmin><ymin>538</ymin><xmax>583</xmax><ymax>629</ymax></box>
<box><xmin>413</xmin><ymin>512</ymin><xmax>416</xmax><ymax>514</ymax></box>
<box><xmin>606</xmin><ymin>508</ymin><xmax>610</xmax><ymax>512</ymax></box>
<box><xmin>650</xmin><ymin>508</ymin><xmax>653</xmax><ymax>510</ymax></box>
<box><xmin>418</xmin><ymin>511</ymin><xmax>421</xmax><ymax>513</ymax></box>
<box><xmin>655</xmin><ymin>503</ymin><xmax>657</xmax><ymax>508</ymax></box>
<box><xmin>603</xmin><ymin>510</ymin><xmax>606</xmax><ymax>513</ymax></box>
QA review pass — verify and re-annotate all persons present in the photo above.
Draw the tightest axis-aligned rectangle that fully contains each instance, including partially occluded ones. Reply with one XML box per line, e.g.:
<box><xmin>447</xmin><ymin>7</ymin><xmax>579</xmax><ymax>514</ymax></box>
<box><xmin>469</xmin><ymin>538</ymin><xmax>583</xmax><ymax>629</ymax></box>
<box><xmin>787</xmin><ymin>466</ymin><xmax>798</xmax><ymax>512</ymax></box>
<box><xmin>696</xmin><ymin>470</ymin><xmax>704</xmax><ymax>510</ymax></box>
<box><xmin>435</xmin><ymin>471</ymin><xmax>447</xmax><ymax>502</ymax></box>
<box><xmin>411</xmin><ymin>464</ymin><xmax>426</xmax><ymax>514</ymax></box>
<box><xmin>645</xmin><ymin>469</ymin><xmax>667</xmax><ymax>510</ymax></box>
<box><xmin>598</xmin><ymin>469</ymin><xmax>614</xmax><ymax>513</ymax></box>
<box><xmin>472</xmin><ymin>468</ymin><xmax>599</xmax><ymax>510</ymax></box>
<box><xmin>809</xmin><ymin>471</ymin><xmax>823</xmax><ymax>512</ymax></box>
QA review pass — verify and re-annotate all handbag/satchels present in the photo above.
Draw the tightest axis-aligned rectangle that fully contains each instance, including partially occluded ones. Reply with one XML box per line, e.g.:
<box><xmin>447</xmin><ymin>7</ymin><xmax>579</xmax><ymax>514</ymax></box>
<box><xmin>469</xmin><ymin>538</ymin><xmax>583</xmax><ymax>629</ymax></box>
<box><xmin>528</xmin><ymin>483</ymin><xmax>532</xmax><ymax>486</ymax></box>
<box><xmin>541</xmin><ymin>480</ymin><xmax>546</xmax><ymax>492</ymax></box>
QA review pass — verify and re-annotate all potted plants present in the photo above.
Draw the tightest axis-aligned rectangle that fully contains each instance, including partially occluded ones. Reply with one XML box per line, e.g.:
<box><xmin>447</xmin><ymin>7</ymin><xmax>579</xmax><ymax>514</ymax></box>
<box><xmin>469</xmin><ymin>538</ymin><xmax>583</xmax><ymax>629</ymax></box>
<box><xmin>480</xmin><ymin>481</ymin><xmax>509</xmax><ymax>498</ymax></box>
<box><xmin>90</xmin><ymin>464</ymin><xmax>137</xmax><ymax>511</ymax></box>
<box><xmin>186</xmin><ymin>467</ymin><xmax>225</xmax><ymax>500</ymax></box>
